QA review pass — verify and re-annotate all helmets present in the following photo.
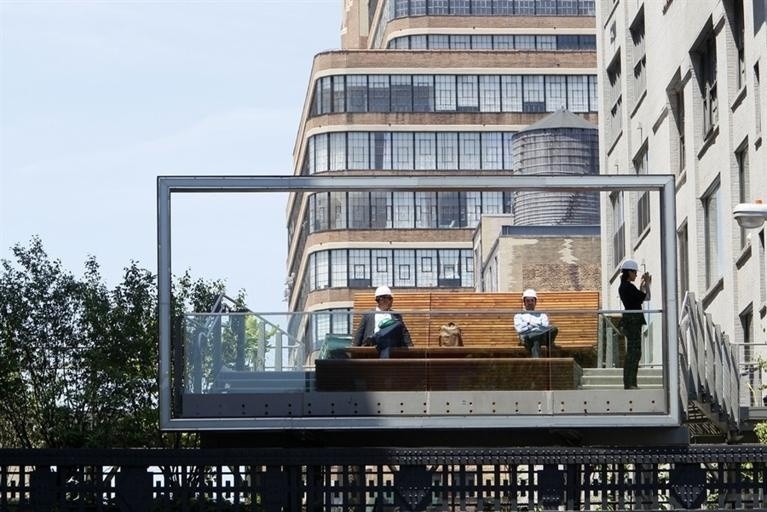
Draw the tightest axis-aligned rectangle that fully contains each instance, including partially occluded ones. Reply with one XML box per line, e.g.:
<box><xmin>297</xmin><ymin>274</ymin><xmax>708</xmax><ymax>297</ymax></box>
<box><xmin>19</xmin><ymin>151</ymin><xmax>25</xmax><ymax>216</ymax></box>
<box><xmin>374</xmin><ymin>285</ymin><xmax>393</xmax><ymax>298</ymax></box>
<box><xmin>619</xmin><ymin>259</ymin><xmax>640</xmax><ymax>273</ymax></box>
<box><xmin>520</xmin><ymin>288</ymin><xmax>539</xmax><ymax>301</ymax></box>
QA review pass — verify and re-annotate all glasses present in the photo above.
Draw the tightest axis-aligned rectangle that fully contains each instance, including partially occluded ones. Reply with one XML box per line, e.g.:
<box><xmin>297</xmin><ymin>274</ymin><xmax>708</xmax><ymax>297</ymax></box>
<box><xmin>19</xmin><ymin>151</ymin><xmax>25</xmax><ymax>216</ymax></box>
<box><xmin>381</xmin><ymin>296</ymin><xmax>392</xmax><ymax>301</ymax></box>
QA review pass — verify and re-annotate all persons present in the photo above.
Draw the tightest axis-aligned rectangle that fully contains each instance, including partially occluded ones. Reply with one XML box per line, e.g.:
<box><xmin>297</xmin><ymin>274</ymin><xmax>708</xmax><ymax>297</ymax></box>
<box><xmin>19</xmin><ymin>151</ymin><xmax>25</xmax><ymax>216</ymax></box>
<box><xmin>513</xmin><ymin>288</ymin><xmax>559</xmax><ymax>356</ymax></box>
<box><xmin>352</xmin><ymin>286</ymin><xmax>414</xmax><ymax>350</ymax></box>
<box><xmin>617</xmin><ymin>260</ymin><xmax>652</xmax><ymax>390</ymax></box>
